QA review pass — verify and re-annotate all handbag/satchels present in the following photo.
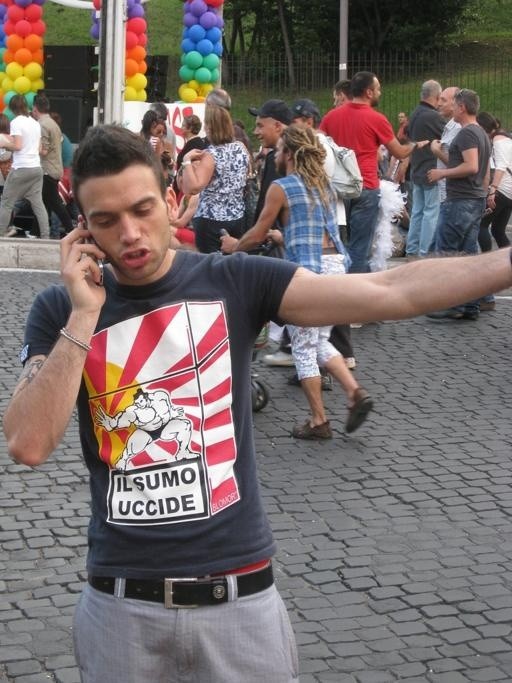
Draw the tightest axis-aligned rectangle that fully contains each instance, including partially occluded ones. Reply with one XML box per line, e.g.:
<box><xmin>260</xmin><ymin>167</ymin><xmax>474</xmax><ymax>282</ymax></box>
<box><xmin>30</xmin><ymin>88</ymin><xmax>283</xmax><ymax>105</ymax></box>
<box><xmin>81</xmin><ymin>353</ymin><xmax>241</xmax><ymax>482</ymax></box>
<box><xmin>330</xmin><ymin>146</ymin><xmax>362</xmax><ymax>200</ymax></box>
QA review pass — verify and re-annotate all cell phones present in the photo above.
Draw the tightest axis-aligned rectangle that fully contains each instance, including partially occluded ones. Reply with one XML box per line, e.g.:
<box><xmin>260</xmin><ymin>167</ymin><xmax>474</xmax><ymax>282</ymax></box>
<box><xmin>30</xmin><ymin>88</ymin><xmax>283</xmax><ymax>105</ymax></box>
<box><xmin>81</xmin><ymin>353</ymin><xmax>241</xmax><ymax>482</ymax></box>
<box><xmin>82</xmin><ymin>221</ymin><xmax>104</xmax><ymax>286</ymax></box>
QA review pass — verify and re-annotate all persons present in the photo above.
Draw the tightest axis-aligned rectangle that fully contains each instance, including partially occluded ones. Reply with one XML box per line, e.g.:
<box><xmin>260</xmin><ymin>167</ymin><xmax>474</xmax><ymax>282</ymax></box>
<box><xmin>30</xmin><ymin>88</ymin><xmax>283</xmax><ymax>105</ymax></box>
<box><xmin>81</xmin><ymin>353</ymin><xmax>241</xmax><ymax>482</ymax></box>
<box><xmin>94</xmin><ymin>387</ymin><xmax>201</xmax><ymax>472</ymax></box>
<box><xmin>136</xmin><ymin>73</ymin><xmax>512</xmax><ymax>438</ymax></box>
<box><xmin>2</xmin><ymin>122</ymin><xmax>511</xmax><ymax>683</ymax></box>
<box><xmin>1</xmin><ymin>93</ymin><xmax>82</xmax><ymax>240</ymax></box>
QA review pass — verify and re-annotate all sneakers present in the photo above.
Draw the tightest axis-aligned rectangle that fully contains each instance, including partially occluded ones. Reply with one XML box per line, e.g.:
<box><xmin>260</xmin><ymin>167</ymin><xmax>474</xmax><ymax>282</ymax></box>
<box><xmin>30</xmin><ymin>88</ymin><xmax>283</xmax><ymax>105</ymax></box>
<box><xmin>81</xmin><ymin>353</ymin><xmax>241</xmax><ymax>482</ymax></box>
<box><xmin>427</xmin><ymin>298</ymin><xmax>496</xmax><ymax>319</ymax></box>
<box><xmin>292</xmin><ymin>420</ymin><xmax>335</xmax><ymax>441</ymax></box>
<box><xmin>264</xmin><ymin>351</ymin><xmax>294</xmax><ymax>367</ymax></box>
<box><xmin>289</xmin><ymin>373</ymin><xmax>334</xmax><ymax>390</ymax></box>
<box><xmin>345</xmin><ymin>388</ymin><xmax>373</xmax><ymax>433</ymax></box>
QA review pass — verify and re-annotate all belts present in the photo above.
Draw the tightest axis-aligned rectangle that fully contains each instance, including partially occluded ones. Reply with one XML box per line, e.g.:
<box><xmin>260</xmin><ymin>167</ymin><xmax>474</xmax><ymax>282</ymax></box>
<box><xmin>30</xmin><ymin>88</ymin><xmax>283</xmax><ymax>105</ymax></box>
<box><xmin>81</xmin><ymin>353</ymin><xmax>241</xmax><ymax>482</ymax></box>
<box><xmin>84</xmin><ymin>566</ymin><xmax>277</xmax><ymax>606</ymax></box>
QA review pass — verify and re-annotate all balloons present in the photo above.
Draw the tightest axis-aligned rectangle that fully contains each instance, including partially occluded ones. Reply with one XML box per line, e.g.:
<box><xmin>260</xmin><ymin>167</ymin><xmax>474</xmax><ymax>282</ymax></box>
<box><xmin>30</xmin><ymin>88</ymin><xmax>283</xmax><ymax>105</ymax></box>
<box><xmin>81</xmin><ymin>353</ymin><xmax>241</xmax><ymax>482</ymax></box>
<box><xmin>0</xmin><ymin>0</ymin><xmax>48</xmax><ymax>121</ymax></box>
<box><xmin>91</xmin><ymin>0</ymin><xmax>149</xmax><ymax>101</ymax></box>
<box><xmin>177</xmin><ymin>0</ymin><xmax>225</xmax><ymax>103</ymax></box>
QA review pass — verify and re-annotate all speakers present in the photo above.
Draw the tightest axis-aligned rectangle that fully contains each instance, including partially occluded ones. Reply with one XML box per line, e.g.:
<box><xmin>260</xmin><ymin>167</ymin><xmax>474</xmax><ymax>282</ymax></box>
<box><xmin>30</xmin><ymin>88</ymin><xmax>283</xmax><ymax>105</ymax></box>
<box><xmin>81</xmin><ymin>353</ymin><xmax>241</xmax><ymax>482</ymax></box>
<box><xmin>38</xmin><ymin>90</ymin><xmax>97</xmax><ymax>143</ymax></box>
<box><xmin>144</xmin><ymin>55</ymin><xmax>168</xmax><ymax>102</ymax></box>
<box><xmin>44</xmin><ymin>46</ymin><xmax>98</xmax><ymax>89</ymax></box>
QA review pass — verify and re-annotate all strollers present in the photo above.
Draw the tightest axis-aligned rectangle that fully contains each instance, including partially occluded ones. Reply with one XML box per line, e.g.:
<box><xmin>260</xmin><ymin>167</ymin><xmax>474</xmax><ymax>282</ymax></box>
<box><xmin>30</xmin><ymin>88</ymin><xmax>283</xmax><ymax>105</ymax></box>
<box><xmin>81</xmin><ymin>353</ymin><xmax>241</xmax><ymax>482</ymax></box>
<box><xmin>220</xmin><ymin>227</ymin><xmax>276</xmax><ymax>412</ymax></box>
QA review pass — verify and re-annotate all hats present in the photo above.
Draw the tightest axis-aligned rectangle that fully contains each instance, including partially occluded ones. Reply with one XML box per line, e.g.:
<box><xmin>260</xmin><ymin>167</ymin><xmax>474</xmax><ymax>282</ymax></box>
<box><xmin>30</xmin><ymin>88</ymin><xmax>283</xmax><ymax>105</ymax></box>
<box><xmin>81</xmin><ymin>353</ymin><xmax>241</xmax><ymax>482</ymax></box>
<box><xmin>287</xmin><ymin>99</ymin><xmax>319</xmax><ymax>120</ymax></box>
<box><xmin>248</xmin><ymin>99</ymin><xmax>291</xmax><ymax>126</ymax></box>
